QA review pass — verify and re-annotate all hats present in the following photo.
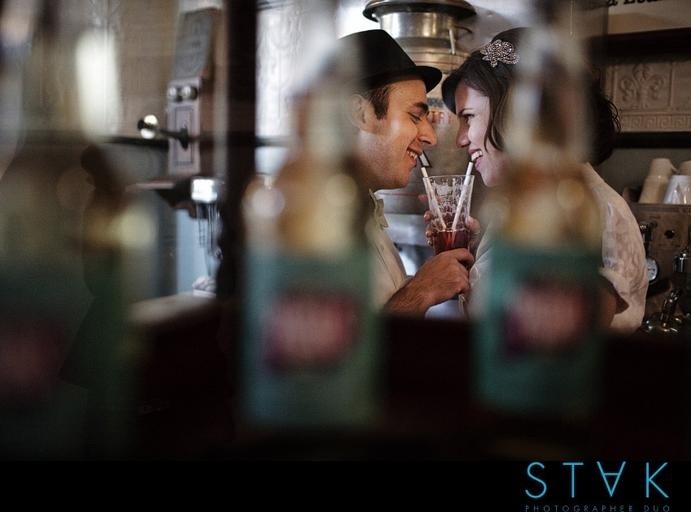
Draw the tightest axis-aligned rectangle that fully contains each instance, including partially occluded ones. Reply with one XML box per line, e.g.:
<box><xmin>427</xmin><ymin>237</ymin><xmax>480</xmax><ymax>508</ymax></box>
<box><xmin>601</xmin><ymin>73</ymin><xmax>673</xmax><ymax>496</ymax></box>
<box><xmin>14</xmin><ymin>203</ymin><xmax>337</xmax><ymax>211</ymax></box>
<box><xmin>337</xmin><ymin>30</ymin><xmax>442</xmax><ymax>94</ymax></box>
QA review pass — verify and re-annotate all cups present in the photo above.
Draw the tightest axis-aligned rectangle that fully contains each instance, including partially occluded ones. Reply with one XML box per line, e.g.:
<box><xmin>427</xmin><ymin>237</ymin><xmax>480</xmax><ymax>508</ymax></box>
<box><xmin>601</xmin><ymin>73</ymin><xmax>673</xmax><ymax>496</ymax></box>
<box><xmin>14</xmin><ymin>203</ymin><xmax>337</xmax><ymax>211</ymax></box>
<box><xmin>422</xmin><ymin>176</ymin><xmax>476</xmax><ymax>254</ymax></box>
<box><xmin>640</xmin><ymin>159</ymin><xmax>691</xmax><ymax>205</ymax></box>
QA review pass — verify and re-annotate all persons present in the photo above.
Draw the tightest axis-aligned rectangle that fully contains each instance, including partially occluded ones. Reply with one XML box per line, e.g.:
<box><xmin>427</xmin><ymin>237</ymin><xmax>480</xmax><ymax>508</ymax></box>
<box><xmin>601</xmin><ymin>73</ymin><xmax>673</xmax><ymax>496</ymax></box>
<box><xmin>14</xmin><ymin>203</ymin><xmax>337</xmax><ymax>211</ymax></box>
<box><xmin>269</xmin><ymin>26</ymin><xmax>649</xmax><ymax>357</ymax></box>
